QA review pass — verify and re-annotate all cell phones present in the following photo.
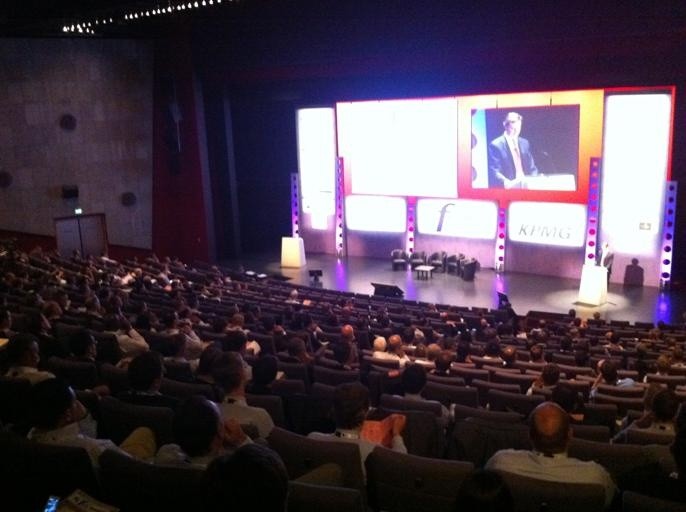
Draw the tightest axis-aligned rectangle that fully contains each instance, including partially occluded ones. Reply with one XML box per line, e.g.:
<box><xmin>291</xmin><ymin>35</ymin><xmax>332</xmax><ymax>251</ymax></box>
<box><xmin>42</xmin><ymin>496</ymin><xmax>60</xmax><ymax>512</ymax></box>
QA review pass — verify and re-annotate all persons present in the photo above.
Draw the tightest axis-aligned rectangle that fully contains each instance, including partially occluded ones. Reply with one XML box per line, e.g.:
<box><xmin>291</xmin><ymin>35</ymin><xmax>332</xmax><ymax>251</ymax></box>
<box><xmin>205</xmin><ymin>445</ymin><xmax>292</xmax><ymax>511</ymax></box>
<box><xmin>488</xmin><ymin>112</ymin><xmax>539</xmax><ymax>188</ymax></box>
<box><xmin>25</xmin><ymin>379</ymin><xmax>155</xmax><ymax>462</ymax></box>
<box><xmin>155</xmin><ymin>395</ymin><xmax>269</xmax><ymax>471</ymax></box>
<box><xmin>307</xmin><ymin>382</ymin><xmax>408</xmax><ymax>486</ymax></box>
<box><xmin>482</xmin><ymin>401</ymin><xmax>616</xmax><ymax>507</ymax></box>
<box><xmin>456</xmin><ymin>468</ymin><xmax>516</xmax><ymax>511</ymax></box>
<box><xmin>0</xmin><ymin>235</ymin><xmax>686</xmax><ymax>438</ymax></box>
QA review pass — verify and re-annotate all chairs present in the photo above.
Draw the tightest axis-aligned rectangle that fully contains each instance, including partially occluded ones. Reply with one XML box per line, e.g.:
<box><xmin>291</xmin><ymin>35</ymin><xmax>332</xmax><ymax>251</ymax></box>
<box><xmin>0</xmin><ymin>248</ymin><xmax>686</xmax><ymax>512</ymax></box>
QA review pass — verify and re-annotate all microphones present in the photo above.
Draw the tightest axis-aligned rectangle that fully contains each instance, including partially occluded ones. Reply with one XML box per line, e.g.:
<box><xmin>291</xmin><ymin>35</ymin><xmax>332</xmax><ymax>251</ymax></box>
<box><xmin>544</xmin><ymin>151</ymin><xmax>556</xmax><ymax>175</ymax></box>
